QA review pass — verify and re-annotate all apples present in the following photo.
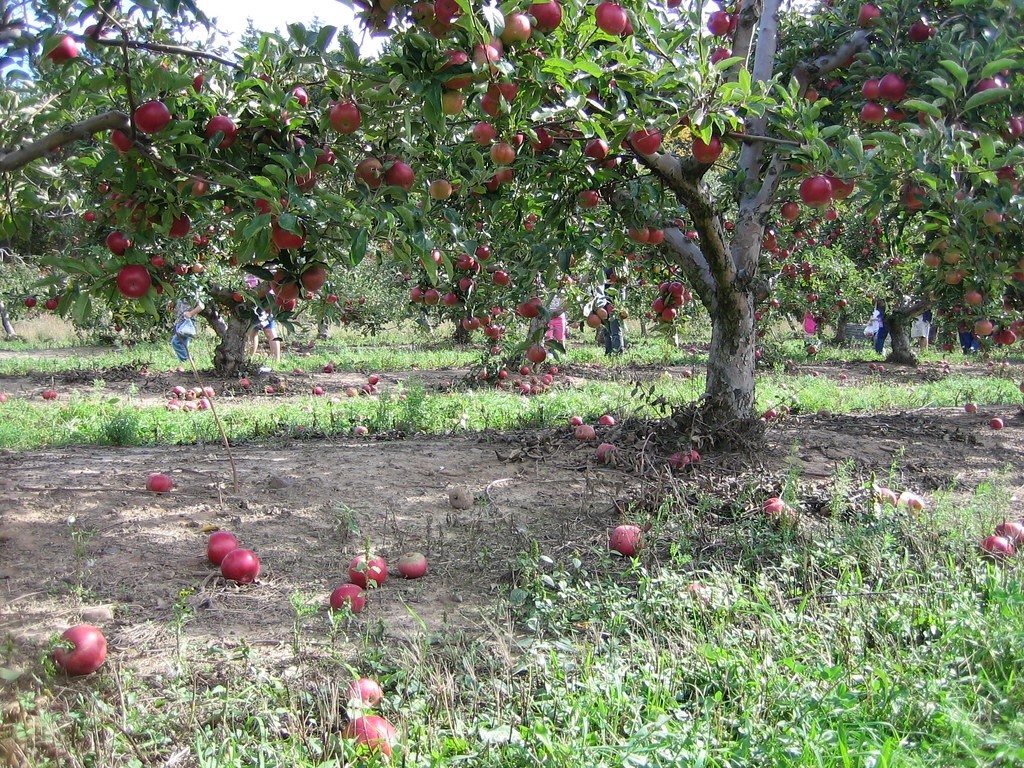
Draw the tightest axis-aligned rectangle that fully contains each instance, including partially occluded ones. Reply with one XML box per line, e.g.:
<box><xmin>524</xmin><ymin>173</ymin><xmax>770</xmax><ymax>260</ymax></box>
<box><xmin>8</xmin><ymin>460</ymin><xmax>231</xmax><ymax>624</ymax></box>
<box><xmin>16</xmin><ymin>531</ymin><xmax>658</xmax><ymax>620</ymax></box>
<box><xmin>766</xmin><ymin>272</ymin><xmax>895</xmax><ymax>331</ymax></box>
<box><xmin>25</xmin><ymin>0</ymin><xmax>1024</xmax><ymax>757</ymax></box>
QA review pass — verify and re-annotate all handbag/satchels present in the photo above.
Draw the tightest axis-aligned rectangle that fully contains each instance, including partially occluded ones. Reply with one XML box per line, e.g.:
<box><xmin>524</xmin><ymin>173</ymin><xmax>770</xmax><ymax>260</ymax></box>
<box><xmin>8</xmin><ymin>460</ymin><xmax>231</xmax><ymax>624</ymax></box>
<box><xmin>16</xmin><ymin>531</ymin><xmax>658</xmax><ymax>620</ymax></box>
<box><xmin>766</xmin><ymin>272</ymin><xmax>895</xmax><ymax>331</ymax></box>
<box><xmin>177</xmin><ymin>312</ymin><xmax>197</xmax><ymax>338</ymax></box>
<box><xmin>864</xmin><ymin>324</ymin><xmax>874</xmax><ymax>337</ymax></box>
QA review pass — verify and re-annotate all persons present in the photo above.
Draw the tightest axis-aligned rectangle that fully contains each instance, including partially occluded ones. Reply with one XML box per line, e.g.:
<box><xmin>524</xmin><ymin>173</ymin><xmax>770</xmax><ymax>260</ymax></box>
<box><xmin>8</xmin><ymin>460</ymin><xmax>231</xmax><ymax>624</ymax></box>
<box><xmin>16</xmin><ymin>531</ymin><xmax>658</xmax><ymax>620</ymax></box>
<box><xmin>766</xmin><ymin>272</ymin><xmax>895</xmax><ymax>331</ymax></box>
<box><xmin>243</xmin><ymin>259</ymin><xmax>281</xmax><ymax>364</ymax></box>
<box><xmin>599</xmin><ymin>254</ymin><xmax>629</xmax><ymax>356</ymax></box>
<box><xmin>167</xmin><ymin>280</ymin><xmax>205</xmax><ymax>362</ymax></box>
<box><xmin>544</xmin><ymin>287</ymin><xmax>567</xmax><ymax>355</ymax></box>
<box><xmin>872</xmin><ymin>293</ymin><xmax>979</xmax><ymax>356</ymax></box>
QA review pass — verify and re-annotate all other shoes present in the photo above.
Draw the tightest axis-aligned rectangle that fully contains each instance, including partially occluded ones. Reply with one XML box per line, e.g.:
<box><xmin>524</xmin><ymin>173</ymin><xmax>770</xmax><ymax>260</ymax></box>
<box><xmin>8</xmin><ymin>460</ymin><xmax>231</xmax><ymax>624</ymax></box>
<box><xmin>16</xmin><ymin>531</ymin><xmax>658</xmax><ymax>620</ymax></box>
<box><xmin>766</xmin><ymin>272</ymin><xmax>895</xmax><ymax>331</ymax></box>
<box><xmin>260</xmin><ymin>366</ymin><xmax>272</xmax><ymax>373</ymax></box>
